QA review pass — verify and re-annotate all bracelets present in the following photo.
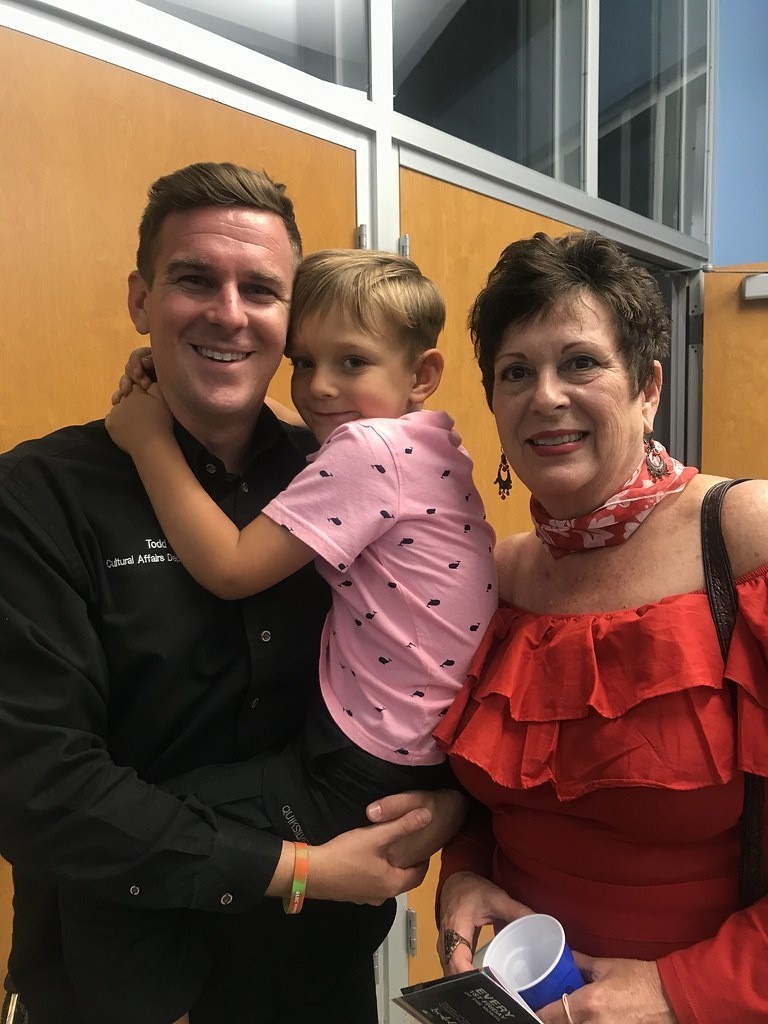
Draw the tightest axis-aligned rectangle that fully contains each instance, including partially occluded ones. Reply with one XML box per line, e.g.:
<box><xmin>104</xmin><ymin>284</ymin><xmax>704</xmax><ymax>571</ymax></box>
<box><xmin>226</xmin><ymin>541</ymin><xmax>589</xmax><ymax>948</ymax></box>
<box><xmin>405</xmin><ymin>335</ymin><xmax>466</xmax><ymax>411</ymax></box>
<box><xmin>284</xmin><ymin>842</ymin><xmax>308</xmax><ymax>914</ymax></box>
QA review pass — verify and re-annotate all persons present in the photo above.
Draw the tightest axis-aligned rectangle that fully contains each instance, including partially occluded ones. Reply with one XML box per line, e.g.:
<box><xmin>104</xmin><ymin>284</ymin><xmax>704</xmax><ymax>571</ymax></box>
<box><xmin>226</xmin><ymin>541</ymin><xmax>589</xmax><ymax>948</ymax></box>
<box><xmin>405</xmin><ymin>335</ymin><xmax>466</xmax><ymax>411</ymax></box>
<box><xmin>106</xmin><ymin>251</ymin><xmax>498</xmax><ymax>1024</ymax></box>
<box><xmin>436</xmin><ymin>236</ymin><xmax>768</xmax><ymax>1024</ymax></box>
<box><xmin>1</xmin><ymin>163</ymin><xmax>467</xmax><ymax>1024</ymax></box>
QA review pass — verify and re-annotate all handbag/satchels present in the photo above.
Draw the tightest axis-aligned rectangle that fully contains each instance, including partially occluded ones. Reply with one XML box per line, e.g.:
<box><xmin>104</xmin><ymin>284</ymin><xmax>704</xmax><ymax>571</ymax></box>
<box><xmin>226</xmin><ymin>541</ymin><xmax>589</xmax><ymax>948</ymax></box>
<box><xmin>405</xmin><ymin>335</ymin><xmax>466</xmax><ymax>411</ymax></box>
<box><xmin>700</xmin><ymin>477</ymin><xmax>768</xmax><ymax>908</ymax></box>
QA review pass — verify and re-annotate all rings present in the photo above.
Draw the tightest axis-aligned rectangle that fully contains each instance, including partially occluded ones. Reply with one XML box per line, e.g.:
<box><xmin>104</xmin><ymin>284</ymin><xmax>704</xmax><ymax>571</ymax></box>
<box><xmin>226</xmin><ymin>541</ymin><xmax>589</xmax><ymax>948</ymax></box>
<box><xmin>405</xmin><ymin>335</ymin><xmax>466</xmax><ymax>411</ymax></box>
<box><xmin>444</xmin><ymin>929</ymin><xmax>474</xmax><ymax>965</ymax></box>
<box><xmin>562</xmin><ymin>993</ymin><xmax>576</xmax><ymax>1023</ymax></box>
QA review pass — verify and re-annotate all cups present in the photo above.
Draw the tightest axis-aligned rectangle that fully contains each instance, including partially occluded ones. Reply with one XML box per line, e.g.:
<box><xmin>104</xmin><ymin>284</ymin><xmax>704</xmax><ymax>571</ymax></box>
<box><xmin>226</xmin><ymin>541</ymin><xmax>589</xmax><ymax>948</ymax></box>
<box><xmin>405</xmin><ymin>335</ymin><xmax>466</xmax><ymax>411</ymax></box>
<box><xmin>481</xmin><ymin>913</ymin><xmax>586</xmax><ymax>1013</ymax></box>
